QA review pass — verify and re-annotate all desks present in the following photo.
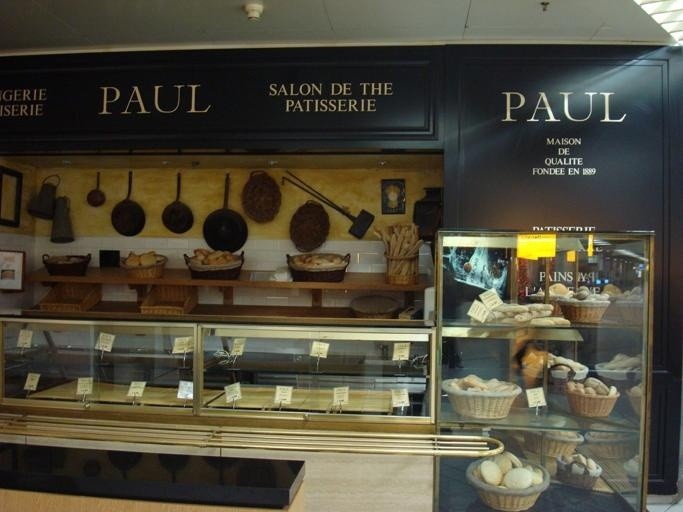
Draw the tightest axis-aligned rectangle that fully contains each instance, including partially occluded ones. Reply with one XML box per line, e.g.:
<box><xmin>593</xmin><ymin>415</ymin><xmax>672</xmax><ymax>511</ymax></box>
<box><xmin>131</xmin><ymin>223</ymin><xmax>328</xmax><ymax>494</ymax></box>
<box><xmin>0</xmin><ymin>442</ymin><xmax>305</xmax><ymax>512</ymax></box>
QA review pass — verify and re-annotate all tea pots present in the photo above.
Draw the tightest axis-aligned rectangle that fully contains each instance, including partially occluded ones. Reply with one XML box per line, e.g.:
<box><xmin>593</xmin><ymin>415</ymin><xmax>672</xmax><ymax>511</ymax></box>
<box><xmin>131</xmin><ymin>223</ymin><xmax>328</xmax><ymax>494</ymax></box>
<box><xmin>24</xmin><ymin>174</ymin><xmax>62</xmax><ymax>220</ymax></box>
<box><xmin>49</xmin><ymin>196</ymin><xmax>73</xmax><ymax>243</ymax></box>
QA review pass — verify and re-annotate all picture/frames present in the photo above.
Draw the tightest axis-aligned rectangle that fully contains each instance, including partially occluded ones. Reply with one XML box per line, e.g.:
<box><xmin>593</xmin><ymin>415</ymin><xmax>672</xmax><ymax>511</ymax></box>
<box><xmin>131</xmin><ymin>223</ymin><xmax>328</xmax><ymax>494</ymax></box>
<box><xmin>0</xmin><ymin>167</ymin><xmax>23</xmax><ymax>227</ymax></box>
<box><xmin>0</xmin><ymin>249</ymin><xmax>26</xmax><ymax>293</ymax></box>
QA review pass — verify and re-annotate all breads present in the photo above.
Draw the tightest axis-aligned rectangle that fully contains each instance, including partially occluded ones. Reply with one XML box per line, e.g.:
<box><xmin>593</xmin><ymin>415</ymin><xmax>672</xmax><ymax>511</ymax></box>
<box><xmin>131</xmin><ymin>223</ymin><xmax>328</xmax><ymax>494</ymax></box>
<box><xmin>560</xmin><ymin>451</ymin><xmax>599</xmax><ymax>470</ymax></box>
<box><xmin>489</xmin><ymin>282</ymin><xmax>608</xmax><ymax>327</ymax></box>
<box><xmin>47</xmin><ymin>256</ymin><xmax>85</xmax><ymax>264</ymax></box>
<box><xmin>605</xmin><ymin>351</ymin><xmax>642</xmax><ymax>370</ymax></box>
<box><xmin>449</xmin><ymin>372</ymin><xmax>512</xmax><ymax>396</ymax></box>
<box><xmin>589</xmin><ymin>431</ymin><xmax>630</xmax><ymax>438</ymax></box>
<box><xmin>544</xmin><ymin>430</ymin><xmax>577</xmax><ymax>439</ymax></box>
<box><xmin>604</xmin><ymin>284</ymin><xmax>641</xmax><ymax>302</ymax></box>
<box><xmin>521</xmin><ymin>351</ymin><xmax>582</xmax><ymax>373</ymax></box>
<box><xmin>627</xmin><ymin>455</ymin><xmax>640</xmax><ymax>470</ymax></box>
<box><xmin>474</xmin><ymin>451</ymin><xmax>543</xmax><ymax>488</ymax></box>
<box><xmin>565</xmin><ymin>377</ymin><xmax>618</xmax><ymax>395</ymax></box>
<box><xmin>186</xmin><ymin>245</ymin><xmax>244</xmax><ymax>269</ymax></box>
<box><xmin>284</xmin><ymin>251</ymin><xmax>348</xmax><ymax>271</ymax></box>
<box><xmin>125</xmin><ymin>251</ymin><xmax>163</xmax><ymax>267</ymax></box>
<box><xmin>630</xmin><ymin>382</ymin><xmax>642</xmax><ymax>395</ymax></box>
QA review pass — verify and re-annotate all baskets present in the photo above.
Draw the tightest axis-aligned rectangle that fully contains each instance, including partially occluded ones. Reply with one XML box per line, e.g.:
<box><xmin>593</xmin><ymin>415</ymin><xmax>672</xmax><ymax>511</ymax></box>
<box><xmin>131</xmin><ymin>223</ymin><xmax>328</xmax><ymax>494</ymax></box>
<box><xmin>595</xmin><ymin>362</ymin><xmax>641</xmax><ymax>418</ymax></box>
<box><xmin>523</xmin><ymin>432</ymin><xmax>583</xmax><ymax>459</ymax></box>
<box><xmin>184</xmin><ymin>251</ymin><xmax>244</xmax><ymax>279</ymax></box>
<box><xmin>566</xmin><ymin>388</ymin><xmax>619</xmax><ymax>418</ymax></box>
<box><xmin>123</xmin><ymin>255</ymin><xmax>168</xmax><ymax>278</ymax></box>
<box><xmin>442</xmin><ymin>378</ymin><xmax>523</xmax><ymax>419</ymax></box>
<box><xmin>584</xmin><ymin>427</ymin><xmax>636</xmax><ymax>459</ymax></box>
<box><xmin>540</xmin><ymin>357</ymin><xmax>590</xmax><ymax>395</ymax></box>
<box><xmin>610</xmin><ymin>295</ymin><xmax>644</xmax><ymax>324</ymax></box>
<box><xmin>526</xmin><ymin>289</ymin><xmax>609</xmax><ymax>322</ymax></box>
<box><xmin>40</xmin><ymin>284</ymin><xmax>101</xmax><ymax>313</ymax></box>
<box><xmin>42</xmin><ymin>254</ymin><xmax>92</xmax><ymax>276</ymax></box>
<box><xmin>286</xmin><ymin>252</ymin><xmax>350</xmax><ymax>282</ymax></box>
<box><xmin>140</xmin><ymin>286</ymin><xmax>198</xmax><ymax>315</ymax></box>
<box><xmin>465</xmin><ymin>457</ymin><xmax>550</xmax><ymax>511</ymax></box>
<box><xmin>556</xmin><ymin>456</ymin><xmax>601</xmax><ymax>490</ymax></box>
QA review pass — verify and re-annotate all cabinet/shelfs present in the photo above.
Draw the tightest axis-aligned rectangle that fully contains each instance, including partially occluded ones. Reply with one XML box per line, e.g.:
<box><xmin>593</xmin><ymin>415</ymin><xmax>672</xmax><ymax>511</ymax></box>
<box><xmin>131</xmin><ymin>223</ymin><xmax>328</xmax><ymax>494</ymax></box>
<box><xmin>433</xmin><ymin>229</ymin><xmax>656</xmax><ymax>512</ymax></box>
<box><xmin>22</xmin><ymin>262</ymin><xmax>429</xmax><ymax>325</ymax></box>
<box><xmin>0</xmin><ymin>315</ymin><xmax>436</xmax><ymax>436</ymax></box>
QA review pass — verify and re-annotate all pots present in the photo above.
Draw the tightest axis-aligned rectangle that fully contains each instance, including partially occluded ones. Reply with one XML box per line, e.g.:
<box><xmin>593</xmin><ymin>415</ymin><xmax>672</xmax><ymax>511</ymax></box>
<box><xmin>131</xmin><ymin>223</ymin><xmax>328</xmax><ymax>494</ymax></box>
<box><xmin>201</xmin><ymin>171</ymin><xmax>248</xmax><ymax>252</ymax></box>
<box><xmin>86</xmin><ymin>173</ymin><xmax>105</xmax><ymax>207</ymax></box>
<box><xmin>161</xmin><ymin>171</ymin><xmax>193</xmax><ymax>234</ymax></box>
<box><xmin>110</xmin><ymin>170</ymin><xmax>145</xmax><ymax>237</ymax></box>
